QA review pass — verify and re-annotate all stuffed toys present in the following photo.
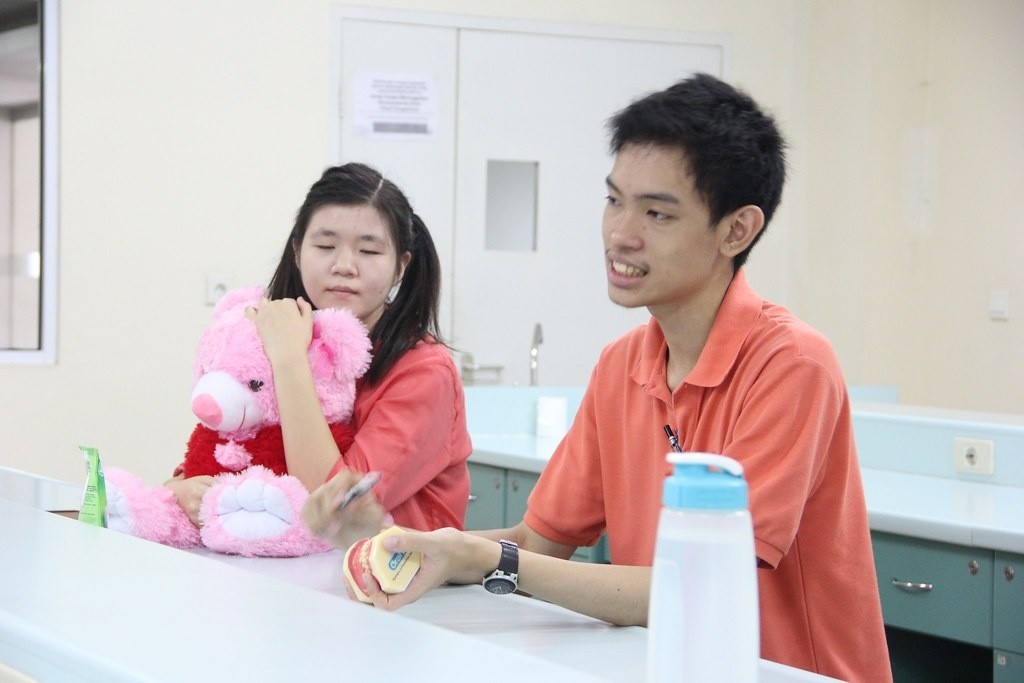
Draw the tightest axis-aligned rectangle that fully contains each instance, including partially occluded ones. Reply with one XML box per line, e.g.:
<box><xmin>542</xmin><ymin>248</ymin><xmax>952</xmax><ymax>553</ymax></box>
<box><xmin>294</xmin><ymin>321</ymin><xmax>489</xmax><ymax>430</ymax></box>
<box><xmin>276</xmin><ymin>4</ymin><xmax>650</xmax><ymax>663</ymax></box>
<box><xmin>101</xmin><ymin>282</ymin><xmax>372</xmax><ymax>559</ymax></box>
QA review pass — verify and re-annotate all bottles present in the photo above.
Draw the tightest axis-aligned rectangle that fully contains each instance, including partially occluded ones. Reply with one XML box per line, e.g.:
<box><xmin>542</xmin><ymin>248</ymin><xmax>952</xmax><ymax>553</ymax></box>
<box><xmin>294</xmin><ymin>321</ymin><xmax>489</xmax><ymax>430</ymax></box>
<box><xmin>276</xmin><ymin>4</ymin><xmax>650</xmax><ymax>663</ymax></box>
<box><xmin>648</xmin><ymin>452</ymin><xmax>760</xmax><ymax>683</ymax></box>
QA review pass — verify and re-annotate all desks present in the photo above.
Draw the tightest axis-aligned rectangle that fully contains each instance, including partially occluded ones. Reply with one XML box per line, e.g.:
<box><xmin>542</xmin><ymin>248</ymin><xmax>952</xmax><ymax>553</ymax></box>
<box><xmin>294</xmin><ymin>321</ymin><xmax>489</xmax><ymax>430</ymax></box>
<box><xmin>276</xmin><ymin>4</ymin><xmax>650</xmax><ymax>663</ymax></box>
<box><xmin>1</xmin><ymin>499</ymin><xmax>845</xmax><ymax>681</ymax></box>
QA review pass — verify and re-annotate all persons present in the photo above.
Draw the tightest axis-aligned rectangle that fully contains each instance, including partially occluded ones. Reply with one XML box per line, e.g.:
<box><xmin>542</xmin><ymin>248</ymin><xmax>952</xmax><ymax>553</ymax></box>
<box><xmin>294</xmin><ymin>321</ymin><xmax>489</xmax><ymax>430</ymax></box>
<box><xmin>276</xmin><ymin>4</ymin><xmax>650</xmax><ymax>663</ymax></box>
<box><xmin>165</xmin><ymin>161</ymin><xmax>474</xmax><ymax>536</ymax></box>
<box><xmin>299</xmin><ymin>70</ymin><xmax>894</xmax><ymax>683</ymax></box>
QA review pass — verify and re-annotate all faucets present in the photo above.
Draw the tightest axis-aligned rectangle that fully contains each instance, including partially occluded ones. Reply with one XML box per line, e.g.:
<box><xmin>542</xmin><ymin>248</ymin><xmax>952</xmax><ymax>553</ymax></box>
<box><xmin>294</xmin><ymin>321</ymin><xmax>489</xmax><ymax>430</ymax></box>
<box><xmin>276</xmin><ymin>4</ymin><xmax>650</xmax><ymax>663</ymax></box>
<box><xmin>528</xmin><ymin>321</ymin><xmax>544</xmax><ymax>385</ymax></box>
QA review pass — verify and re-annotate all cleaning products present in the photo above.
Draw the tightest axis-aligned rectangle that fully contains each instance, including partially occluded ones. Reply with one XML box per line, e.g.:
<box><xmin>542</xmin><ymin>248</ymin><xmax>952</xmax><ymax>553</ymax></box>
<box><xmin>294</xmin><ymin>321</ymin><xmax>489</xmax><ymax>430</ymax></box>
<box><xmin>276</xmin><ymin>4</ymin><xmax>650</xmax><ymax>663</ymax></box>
<box><xmin>74</xmin><ymin>444</ymin><xmax>108</xmax><ymax>529</ymax></box>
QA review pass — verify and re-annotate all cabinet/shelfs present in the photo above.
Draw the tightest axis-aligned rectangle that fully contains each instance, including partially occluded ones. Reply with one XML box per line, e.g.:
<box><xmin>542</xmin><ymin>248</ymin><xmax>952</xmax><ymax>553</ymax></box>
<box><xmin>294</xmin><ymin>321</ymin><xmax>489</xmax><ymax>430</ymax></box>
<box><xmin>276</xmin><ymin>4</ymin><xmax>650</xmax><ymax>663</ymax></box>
<box><xmin>464</xmin><ymin>432</ymin><xmax>1023</xmax><ymax>683</ymax></box>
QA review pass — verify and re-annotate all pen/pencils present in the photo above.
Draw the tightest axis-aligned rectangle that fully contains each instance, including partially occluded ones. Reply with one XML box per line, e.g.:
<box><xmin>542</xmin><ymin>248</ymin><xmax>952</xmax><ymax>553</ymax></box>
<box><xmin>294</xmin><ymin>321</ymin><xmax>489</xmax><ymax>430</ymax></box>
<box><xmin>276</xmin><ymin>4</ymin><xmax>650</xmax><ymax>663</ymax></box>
<box><xmin>334</xmin><ymin>469</ymin><xmax>383</xmax><ymax>510</ymax></box>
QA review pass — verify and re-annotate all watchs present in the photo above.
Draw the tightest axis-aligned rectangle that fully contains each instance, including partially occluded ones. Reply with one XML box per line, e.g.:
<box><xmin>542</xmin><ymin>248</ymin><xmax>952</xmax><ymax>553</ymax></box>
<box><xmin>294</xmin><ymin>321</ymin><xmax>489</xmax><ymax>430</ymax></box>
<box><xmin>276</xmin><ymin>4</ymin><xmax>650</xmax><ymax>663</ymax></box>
<box><xmin>481</xmin><ymin>539</ymin><xmax>518</xmax><ymax>595</ymax></box>
